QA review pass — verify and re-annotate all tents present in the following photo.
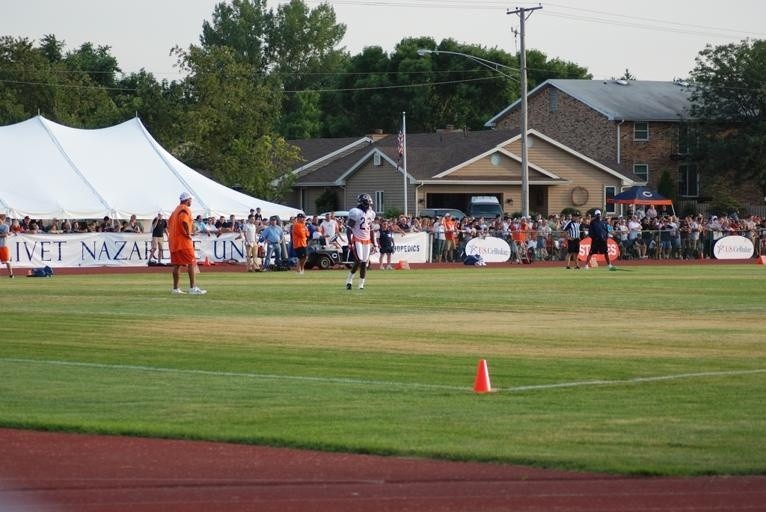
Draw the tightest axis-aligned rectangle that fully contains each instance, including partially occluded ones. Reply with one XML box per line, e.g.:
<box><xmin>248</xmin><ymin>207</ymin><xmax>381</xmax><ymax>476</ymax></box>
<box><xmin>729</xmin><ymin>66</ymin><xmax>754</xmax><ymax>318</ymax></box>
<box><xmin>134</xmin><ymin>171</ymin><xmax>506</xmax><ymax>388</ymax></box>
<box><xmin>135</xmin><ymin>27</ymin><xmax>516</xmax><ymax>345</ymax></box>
<box><xmin>608</xmin><ymin>186</ymin><xmax>676</xmax><ymax>218</ymax></box>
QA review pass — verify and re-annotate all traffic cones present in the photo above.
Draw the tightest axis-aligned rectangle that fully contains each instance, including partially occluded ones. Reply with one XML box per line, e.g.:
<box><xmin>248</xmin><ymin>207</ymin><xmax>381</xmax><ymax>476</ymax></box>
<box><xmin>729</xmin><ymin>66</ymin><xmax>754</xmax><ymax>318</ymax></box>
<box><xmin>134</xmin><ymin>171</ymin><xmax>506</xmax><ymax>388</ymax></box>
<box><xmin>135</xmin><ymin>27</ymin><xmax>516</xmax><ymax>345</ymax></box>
<box><xmin>473</xmin><ymin>359</ymin><xmax>490</xmax><ymax>393</ymax></box>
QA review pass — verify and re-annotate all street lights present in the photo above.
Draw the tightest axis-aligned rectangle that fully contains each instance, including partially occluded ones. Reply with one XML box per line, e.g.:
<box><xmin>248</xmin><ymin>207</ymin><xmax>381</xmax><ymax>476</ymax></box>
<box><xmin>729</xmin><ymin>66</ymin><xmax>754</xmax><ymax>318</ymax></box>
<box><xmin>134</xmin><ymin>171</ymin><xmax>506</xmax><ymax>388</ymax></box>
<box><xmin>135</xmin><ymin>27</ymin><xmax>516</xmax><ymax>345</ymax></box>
<box><xmin>418</xmin><ymin>49</ymin><xmax>531</xmax><ymax>219</ymax></box>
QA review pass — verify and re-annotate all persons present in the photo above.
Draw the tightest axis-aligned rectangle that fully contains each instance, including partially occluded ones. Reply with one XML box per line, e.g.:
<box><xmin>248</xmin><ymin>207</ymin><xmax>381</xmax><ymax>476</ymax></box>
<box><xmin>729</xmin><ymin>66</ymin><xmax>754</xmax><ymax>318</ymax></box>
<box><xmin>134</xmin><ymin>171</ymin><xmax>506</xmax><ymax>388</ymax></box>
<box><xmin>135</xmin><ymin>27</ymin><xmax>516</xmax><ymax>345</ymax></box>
<box><xmin>1</xmin><ymin>211</ymin><xmax>168</xmax><ymax>278</ymax></box>
<box><xmin>345</xmin><ymin>193</ymin><xmax>380</xmax><ymax>291</ymax></box>
<box><xmin>370</xmin><ymin>204</ymin><xmax>765</xmax><ymax>272</ymax></box>
<box><xmin>168</xmin><ymin>192</ymin><xmax>206</xmax><ymax>296</ymax></box>
<box><xmin>193</xmin><ymin>206</ymin><xmax>346</xmax><ymax>273</ymax></box>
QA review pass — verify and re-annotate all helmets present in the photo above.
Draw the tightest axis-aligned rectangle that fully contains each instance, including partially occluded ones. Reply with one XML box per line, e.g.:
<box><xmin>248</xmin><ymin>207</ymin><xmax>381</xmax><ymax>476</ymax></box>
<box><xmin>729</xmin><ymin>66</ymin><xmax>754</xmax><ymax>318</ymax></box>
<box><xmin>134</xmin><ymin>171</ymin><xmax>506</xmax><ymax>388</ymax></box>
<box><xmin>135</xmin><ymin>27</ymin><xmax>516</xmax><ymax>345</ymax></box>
<box><xmin>357</xmin><ymin>194</ymin><xmax>374</xmax><ymax>207</ymax></box>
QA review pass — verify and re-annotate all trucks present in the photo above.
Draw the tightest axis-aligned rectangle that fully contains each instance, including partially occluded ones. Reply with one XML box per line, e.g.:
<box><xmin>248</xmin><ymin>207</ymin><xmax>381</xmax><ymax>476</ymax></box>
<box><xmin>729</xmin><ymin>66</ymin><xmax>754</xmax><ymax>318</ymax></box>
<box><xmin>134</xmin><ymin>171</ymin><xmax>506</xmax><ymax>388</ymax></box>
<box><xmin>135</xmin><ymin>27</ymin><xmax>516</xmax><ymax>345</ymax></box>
<box><xmin>466</xmin><ymin>196</ymin><xmax>504</xmax><ymax>222</ymax></box>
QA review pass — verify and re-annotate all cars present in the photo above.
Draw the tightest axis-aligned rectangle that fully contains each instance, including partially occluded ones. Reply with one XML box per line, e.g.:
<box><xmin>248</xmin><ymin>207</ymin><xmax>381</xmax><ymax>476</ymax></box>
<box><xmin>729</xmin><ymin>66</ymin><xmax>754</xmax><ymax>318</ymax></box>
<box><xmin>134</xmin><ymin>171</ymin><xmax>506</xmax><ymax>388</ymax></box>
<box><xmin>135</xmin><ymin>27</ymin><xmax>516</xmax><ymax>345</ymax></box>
<box><xmin>419</xmin><ymin>208</ymin><xmax>467</xmax><ymax>222</ymax></box>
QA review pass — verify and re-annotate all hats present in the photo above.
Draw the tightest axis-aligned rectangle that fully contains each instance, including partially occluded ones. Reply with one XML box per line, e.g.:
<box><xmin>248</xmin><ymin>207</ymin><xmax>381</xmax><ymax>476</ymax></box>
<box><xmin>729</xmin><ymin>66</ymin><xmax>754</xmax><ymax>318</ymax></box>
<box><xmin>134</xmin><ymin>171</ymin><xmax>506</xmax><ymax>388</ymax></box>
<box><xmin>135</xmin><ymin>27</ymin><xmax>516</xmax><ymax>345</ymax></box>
<box><xmin>595</xmin><ymin>209</ymin><xmax>601</xmax><ymax>215</ymax></box>
<box><xmin>179</xmin><ymin>192</ymin><xmax>191</xmax><ymax>201</ymax></box>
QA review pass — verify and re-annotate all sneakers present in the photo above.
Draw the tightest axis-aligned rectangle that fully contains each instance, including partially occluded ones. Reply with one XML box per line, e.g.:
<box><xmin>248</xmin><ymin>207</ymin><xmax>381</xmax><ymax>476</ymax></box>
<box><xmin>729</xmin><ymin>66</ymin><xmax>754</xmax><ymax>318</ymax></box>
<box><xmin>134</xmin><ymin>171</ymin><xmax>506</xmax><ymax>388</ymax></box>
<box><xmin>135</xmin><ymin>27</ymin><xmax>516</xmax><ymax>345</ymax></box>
<box><xmin>189</xmin><ymin>287</ymin><xmax>207</xmax><ymax>295</ymax></box>
<box><xmin>171</xmin><ymin>289</ymin><xmax>186</xmax><ymax>294</ymax></box>
<box><xmin>346</xmin><ymin>283</ymin><xmax>351</xmax><ymax>289</ymax></box>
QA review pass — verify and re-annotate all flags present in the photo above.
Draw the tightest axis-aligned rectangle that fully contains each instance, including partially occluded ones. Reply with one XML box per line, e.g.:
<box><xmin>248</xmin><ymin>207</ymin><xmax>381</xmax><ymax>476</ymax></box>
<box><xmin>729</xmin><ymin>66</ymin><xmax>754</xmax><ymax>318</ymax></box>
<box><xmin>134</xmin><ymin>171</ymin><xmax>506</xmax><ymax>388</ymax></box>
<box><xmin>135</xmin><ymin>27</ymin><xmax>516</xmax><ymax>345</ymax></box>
<box><xmin>396</xmin><ymin>118</ymin><xmax>403</xmax><ymax>172</ymax></box>
